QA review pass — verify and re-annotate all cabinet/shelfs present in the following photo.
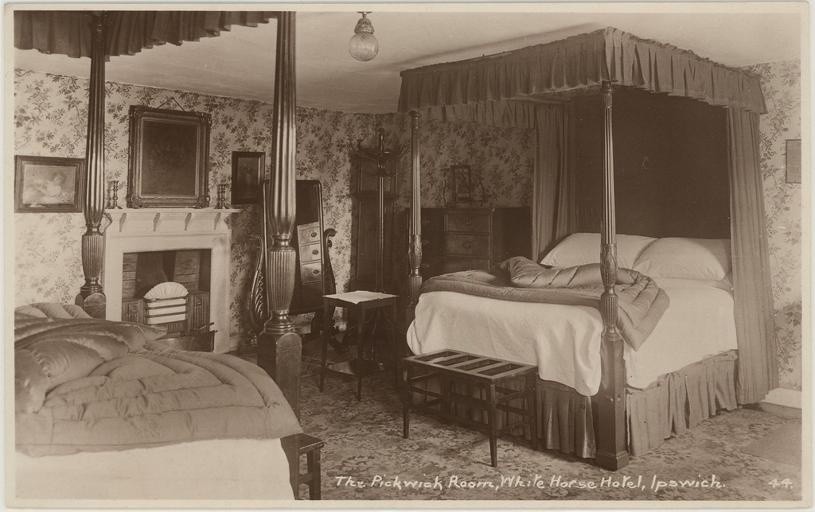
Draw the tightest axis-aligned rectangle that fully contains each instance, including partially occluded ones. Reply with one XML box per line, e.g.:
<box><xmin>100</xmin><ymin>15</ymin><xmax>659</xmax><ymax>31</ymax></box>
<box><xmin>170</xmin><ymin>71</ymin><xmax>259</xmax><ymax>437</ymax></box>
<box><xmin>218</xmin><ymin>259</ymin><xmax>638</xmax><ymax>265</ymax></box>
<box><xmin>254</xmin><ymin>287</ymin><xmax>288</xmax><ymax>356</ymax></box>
<box><xmin>294</xmin><ymin>217</ymin><xmax>323</xmax><ymax>306</ymax></box>
<box><xmin>403</xmin><ymin>202</ymin><xmax>535</xmax><ymax>285</ymax></box>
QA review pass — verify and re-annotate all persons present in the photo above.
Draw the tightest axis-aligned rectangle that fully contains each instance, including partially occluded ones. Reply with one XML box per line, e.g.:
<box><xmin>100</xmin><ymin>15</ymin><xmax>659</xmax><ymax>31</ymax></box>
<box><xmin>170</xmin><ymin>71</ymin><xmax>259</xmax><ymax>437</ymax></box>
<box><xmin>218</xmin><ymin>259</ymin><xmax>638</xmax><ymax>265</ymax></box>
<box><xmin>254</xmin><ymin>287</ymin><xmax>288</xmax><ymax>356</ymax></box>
<box><xmin>32</xmin><ymin>169</ymin><xmax>70</xmax><ymax>200</ymax></box>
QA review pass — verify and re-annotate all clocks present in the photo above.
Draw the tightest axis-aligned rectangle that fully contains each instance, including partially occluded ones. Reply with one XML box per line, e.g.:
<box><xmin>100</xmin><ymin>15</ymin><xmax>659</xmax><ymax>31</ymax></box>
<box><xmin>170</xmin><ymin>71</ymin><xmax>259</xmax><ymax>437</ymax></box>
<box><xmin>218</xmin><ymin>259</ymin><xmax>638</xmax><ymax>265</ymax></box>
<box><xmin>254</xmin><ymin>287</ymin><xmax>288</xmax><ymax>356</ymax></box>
<box><xmin>355</xmin><ymin>146</ymin><xmax>398</xmax><ymax>201</ymax></box>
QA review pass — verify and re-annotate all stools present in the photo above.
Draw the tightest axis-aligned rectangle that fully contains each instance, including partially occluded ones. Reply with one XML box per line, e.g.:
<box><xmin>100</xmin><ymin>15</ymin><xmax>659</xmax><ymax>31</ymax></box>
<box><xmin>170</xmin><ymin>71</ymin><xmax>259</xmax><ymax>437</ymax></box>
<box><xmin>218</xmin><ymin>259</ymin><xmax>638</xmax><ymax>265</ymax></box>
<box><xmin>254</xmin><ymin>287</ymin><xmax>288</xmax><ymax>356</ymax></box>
<box><xmin>396</xmin><ymin>348</ymin><xmax>542</xmax><ymax>467</ymax></box>
<box><xmin>313</xmin><ymin>290</ymin><xmax>406</xmax><ymax>402</ymax></box>
<box><xmin>278</xmin><ymin>430</ymin><xmax>327</xmax><ymax>499</ymax></box>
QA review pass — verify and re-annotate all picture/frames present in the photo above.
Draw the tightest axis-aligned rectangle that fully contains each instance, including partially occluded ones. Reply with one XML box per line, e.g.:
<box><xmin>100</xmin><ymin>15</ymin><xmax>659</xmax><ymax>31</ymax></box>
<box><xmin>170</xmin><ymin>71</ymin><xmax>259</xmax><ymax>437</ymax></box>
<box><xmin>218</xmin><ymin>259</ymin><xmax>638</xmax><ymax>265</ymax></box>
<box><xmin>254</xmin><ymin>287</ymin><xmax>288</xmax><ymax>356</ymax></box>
<box><xmin>12</xmin><ymin>151</ymin><xmax>91</xmax><ymax>215</ymax></box>
<box><xmin>124</xmin><ymin>104</ymin><xmax>216</xmax><ymax>210</ymax></box>
<box><xmin>229</xmin><ymin>149</ymin><xmax>268</xmax><ymax>207</ymax></box>
<box><xmin>448</xmin><ymin>163</ymin><xmax>473</xmax><ymax>206</ymax></box>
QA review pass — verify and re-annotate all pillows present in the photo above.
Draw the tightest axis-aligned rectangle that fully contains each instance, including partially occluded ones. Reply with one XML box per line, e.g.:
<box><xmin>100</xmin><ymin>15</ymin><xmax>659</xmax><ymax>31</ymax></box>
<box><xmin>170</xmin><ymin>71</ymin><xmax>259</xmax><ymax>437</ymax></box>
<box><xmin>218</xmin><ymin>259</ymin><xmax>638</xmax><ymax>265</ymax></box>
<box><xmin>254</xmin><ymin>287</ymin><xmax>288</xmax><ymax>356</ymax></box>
<box><xmin>632</xmin><ymin>236</ymin><xmax>739</xmax><ymax>283</ymax></box>
<box><xmin>541</xmin><ymin>229</ymin><xmax>660</xmax><ymax>275</ymax></box>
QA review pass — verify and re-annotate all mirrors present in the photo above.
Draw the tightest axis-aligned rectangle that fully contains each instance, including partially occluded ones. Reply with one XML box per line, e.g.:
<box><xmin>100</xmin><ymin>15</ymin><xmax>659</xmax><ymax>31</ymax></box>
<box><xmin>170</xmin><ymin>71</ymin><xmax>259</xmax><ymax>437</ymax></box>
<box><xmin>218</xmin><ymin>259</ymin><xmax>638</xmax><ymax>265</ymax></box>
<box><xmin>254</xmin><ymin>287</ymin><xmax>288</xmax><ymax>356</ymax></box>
<box><xmin>261</xmin><ymin>177</ymin><xmax>327</xmax><ymax>318</ymax></box>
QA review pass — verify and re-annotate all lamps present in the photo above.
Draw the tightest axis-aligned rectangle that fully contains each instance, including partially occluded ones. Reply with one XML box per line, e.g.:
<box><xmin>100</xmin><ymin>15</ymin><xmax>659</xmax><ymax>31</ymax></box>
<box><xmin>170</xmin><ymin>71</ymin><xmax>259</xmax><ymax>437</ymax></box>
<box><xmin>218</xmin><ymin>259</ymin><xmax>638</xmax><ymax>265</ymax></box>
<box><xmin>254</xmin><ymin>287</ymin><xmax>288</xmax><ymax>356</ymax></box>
<box><xmin>346</xmin><ymin>12</ymin><xmax>384</xmax><ymax>63</ymax></box>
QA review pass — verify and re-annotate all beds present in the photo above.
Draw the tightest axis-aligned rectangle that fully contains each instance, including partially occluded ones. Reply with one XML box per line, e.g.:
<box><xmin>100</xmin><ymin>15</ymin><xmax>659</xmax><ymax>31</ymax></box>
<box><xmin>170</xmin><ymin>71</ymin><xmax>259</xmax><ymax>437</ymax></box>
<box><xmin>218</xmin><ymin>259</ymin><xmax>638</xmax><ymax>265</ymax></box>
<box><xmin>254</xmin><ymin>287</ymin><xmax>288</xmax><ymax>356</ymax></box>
<box><xmin>11</xmin><ymin>12</ymin><xmax>312</xmax><ymax>499</ymax></box>
<box><xmin>389</xmin><ymin>26</ymin><xmax>786</xmax><ymax>471</ymax></box>
